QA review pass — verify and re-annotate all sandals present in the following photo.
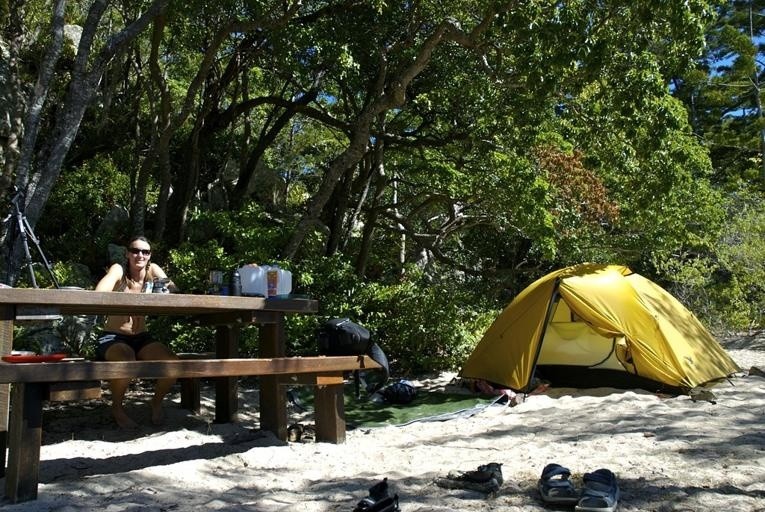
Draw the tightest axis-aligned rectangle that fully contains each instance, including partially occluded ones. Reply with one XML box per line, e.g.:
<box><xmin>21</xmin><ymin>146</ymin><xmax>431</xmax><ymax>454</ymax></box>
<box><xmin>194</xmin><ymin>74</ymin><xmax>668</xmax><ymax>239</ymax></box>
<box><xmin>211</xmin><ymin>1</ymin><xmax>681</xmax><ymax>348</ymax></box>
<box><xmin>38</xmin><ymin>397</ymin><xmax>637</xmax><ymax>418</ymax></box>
<box><xmin>537</xmin><ymin>464</ymin><xmax>581</xmax><ymax>505</ymax></box>
<box><xmin>359</xmin><ymin>477</ymin><xmax>389</xmax><ymax>508</ymax></box>
<box><xmin>353</xmin><ymin>494</ymin><xmax>401</xmax><ymax>512</ymax></box>
<box><xmin>575</xmin><ymin>469</ymin><xmax>621</xmax><ymax>512</ymax></box>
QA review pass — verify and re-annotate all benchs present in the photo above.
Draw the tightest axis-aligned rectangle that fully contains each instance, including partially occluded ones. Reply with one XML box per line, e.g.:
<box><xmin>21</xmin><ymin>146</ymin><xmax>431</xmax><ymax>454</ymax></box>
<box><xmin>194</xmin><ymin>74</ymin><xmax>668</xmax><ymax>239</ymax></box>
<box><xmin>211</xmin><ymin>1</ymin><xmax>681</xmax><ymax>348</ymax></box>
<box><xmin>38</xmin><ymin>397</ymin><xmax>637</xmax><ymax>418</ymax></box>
<box><xmin>11</xmin><ymin>352</ymin><xmax>217</xmax><ymax>413</ymax></box>
<box><xmin>0</xmin><ymin>353</ymin><xmax>383</xmax><ymax>508</ymax></box>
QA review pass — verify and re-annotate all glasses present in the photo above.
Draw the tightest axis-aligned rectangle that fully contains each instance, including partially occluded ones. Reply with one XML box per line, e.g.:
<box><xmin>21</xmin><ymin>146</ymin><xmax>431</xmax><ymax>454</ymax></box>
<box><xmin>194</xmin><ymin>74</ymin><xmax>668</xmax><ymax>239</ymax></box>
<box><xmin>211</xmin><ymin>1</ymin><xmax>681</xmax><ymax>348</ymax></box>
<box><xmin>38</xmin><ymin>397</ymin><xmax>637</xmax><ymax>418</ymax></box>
<box><xmin>126</xmin><ymin>246</ymin><xmax>152</xmax><ymax>256</ymax></box>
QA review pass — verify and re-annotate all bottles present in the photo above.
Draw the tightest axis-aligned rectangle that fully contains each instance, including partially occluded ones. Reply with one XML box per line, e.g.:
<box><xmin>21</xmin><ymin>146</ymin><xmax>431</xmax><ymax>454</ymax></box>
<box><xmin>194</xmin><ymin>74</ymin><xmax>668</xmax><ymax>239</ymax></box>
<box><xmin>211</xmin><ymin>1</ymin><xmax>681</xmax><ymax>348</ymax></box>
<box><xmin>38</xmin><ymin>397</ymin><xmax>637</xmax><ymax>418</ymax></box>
<box><xmin>237</xmin><ymin>263</ymin><xmax>292</xmax><ymax>298</ymax></box>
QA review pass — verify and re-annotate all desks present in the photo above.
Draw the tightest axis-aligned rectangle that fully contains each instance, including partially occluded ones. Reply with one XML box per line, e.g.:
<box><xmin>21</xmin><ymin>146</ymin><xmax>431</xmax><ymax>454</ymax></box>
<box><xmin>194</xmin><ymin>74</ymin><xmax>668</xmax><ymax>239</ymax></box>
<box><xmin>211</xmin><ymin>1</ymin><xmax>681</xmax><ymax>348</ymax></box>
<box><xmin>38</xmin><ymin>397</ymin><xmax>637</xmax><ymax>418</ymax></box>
<box><xmin>0</xmin><ymin>285</ymin><xmax>320</xmax><ymax>446</ymax></box>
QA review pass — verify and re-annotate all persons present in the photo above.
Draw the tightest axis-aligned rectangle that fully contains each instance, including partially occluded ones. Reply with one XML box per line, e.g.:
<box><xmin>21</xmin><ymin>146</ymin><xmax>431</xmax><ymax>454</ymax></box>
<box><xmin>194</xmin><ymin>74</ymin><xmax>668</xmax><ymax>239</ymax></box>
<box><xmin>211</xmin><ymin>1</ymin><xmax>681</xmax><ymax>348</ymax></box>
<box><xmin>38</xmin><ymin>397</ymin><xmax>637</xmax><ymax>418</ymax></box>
<box><xmin>87</xmin><ymin>233</ymin><xmax>184</xmax><ymax>431</ymax></box>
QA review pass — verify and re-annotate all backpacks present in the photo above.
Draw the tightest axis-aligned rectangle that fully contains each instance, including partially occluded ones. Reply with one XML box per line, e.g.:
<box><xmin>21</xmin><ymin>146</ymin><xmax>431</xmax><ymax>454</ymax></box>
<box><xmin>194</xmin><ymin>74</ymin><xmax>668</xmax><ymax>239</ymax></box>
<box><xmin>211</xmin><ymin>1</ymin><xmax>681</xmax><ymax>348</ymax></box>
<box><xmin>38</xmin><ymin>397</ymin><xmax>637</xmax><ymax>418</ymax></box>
<box><xmin>320</xmin><ymin>316</ymin><xmax>390</xmax><ymax>396</ymax></box>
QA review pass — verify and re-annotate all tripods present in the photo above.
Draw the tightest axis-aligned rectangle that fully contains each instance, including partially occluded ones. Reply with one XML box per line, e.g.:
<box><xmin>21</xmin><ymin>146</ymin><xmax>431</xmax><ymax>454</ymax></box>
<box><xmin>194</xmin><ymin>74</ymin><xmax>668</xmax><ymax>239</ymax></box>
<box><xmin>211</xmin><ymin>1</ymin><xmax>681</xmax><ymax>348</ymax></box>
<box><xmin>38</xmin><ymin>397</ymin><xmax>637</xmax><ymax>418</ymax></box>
<box><xmin>0</xmin><ymin>198</ymin><xmax>56</xmax><ymax>287</ymax></box>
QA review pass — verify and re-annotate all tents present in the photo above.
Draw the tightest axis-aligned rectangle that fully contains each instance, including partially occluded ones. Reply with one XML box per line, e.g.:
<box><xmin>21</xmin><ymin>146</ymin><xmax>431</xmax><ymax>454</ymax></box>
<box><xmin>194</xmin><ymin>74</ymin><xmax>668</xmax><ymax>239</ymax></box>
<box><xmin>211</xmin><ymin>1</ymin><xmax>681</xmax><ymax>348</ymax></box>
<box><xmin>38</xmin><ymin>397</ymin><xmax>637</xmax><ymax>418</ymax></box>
<box><xmin>458</xmin><ymin>262</ymin><xmax>749</xmax><ymax>396</ymax></box>
